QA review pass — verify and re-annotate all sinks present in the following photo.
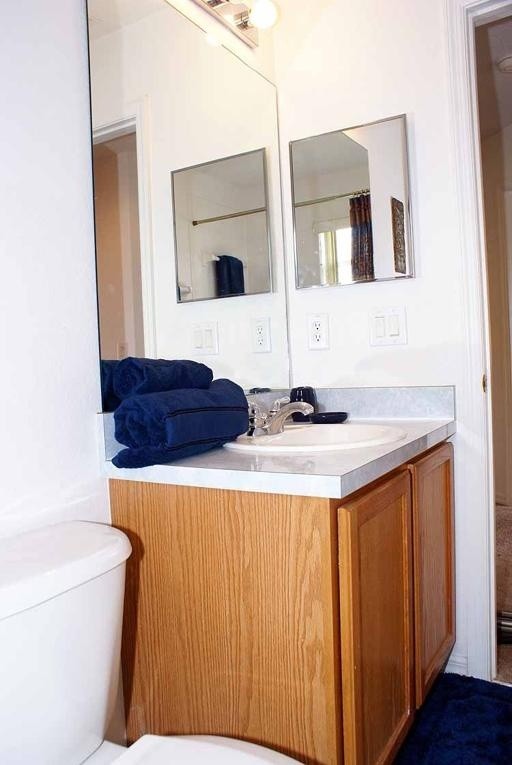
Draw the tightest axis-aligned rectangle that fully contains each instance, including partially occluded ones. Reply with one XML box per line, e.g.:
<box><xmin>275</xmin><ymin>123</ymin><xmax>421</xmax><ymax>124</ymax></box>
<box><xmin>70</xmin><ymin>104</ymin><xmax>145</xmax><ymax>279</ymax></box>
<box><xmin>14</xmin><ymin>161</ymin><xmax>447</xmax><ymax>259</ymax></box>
<box><xmin>223</xmin><ymin>423</ymin><xmax>407</xmax><ymax>453</ymax></box>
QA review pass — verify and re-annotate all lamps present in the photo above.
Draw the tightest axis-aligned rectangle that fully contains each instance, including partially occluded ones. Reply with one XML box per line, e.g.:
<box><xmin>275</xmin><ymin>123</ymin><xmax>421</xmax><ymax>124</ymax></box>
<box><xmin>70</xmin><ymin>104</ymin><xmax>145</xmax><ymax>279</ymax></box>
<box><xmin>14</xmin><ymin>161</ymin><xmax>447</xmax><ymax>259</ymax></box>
<box><xmin>190</xmin><ymin>0</ymin><xmax>281</xmax><ymax>51</ymax></box>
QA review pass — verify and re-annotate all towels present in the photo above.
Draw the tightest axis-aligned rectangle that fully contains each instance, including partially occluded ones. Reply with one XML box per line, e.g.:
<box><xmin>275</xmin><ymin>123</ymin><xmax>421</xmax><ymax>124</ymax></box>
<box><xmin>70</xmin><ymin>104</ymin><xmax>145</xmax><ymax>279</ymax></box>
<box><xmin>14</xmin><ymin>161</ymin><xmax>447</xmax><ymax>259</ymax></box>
<box><xmin>108</xmin><ymin>356</ymin><xmax>251</xmax><ymax>469</ymax></box>
<box><xmin>99</xmin><ymin>355</ymin><xmax>122</xmax><ymax>411</ymax></box>
<box><xmin>214</xmin><ymin>254</ymin><xmax>245</xmax><ymax>299</ymax></box>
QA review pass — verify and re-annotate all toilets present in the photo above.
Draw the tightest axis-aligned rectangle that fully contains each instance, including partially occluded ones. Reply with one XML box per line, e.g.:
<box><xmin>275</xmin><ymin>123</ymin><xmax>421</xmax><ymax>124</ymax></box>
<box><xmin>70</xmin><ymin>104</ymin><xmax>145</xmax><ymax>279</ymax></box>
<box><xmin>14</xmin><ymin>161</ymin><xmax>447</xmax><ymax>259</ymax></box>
<box><xmin>1</xmin><ymin>520</ymin><xmax>306</xmax><ymax>765</ymax></box>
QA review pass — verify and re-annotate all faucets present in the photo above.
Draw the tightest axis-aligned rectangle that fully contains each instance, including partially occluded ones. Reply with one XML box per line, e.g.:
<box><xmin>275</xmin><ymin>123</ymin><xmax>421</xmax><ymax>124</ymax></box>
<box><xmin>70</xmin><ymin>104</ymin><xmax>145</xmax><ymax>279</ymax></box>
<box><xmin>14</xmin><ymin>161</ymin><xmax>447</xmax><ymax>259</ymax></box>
<box><xmin>262</xmin><ymin>401</ymin><xmax>315</xmax><ymax>434</ymax></box>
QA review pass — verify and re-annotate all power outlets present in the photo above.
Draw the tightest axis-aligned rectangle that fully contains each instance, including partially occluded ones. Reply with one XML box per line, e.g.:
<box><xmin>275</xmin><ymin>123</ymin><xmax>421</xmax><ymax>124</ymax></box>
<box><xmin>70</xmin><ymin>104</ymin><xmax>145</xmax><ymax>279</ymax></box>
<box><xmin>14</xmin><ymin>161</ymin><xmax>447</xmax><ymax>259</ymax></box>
<box><xmin>307</xmin><ymin>313</ymin><xmax>329</xmax><ymax>351</ymax></box>
<box><xmin>249</xmin><ymin>316</ymin><xmax>271</xmax><ymax>352</ymax></box>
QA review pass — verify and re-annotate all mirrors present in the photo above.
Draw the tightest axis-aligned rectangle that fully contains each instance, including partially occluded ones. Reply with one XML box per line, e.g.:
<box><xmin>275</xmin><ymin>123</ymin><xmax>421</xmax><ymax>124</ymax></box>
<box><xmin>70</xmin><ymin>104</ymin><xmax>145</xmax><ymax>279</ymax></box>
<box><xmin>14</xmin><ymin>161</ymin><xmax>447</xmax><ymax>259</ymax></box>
<box><xmin>171</xmin><ymin>147</ymin><xmax>271</xmax><ymax>304</ymax></box>
<box><xmin>290</xmin><ymin>114</ymin><xmax>413</xmax><ymax>291</ymax></box>
<box><xmin>84</xmin><ymin>1</ymin><xmax>292</xmax><ymax>416</ymax></box>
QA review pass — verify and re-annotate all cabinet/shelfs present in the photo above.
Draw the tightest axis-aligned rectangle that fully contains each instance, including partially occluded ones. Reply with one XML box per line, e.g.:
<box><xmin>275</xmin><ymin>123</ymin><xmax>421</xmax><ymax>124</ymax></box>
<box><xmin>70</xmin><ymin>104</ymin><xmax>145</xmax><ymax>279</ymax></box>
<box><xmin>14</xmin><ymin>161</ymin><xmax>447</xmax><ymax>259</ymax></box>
<box><xmin>103</xmin><ymin>419</ymin><xmax>454</xmax><ymax>765</ymax></box>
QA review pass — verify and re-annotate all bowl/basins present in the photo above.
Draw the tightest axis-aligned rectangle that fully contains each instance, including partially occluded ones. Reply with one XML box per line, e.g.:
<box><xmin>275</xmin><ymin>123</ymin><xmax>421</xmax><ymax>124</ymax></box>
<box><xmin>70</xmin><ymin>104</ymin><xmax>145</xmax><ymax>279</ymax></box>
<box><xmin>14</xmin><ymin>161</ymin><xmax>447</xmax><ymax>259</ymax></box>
<box><xmin>311</xmin><ymin>411</ymin><xmax>347</xmax><ymax>423</ymax></box>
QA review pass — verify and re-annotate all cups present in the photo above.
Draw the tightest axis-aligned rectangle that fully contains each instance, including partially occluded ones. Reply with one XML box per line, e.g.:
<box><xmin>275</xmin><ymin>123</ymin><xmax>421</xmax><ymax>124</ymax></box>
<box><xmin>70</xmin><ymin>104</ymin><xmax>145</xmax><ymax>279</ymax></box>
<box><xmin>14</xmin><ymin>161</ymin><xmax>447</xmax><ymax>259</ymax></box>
<box><xmin>288</xmin><ymin>386</ymin><xmax>316</xmax><ymax>423</ymax></box>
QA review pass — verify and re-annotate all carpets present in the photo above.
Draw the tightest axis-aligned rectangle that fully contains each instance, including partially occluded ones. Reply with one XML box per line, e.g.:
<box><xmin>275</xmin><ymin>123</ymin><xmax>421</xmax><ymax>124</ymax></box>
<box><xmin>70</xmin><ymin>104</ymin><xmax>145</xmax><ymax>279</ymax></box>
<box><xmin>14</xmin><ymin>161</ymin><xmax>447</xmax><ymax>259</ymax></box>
<box><xmin>393</xmin><ymin>670</ymin><xmax>512</xmax><ymax>764</ymax></box>
